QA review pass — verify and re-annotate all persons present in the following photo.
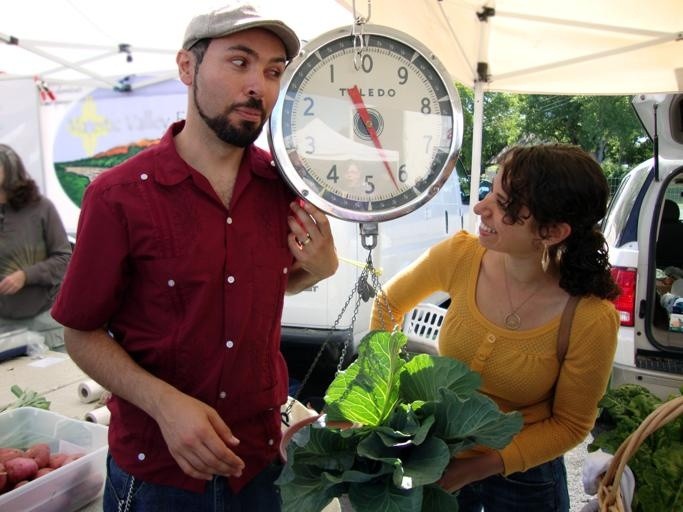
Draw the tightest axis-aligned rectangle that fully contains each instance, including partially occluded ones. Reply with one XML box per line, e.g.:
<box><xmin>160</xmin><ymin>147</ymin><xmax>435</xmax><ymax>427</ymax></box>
<box><xmin>371</xmin><ymin>144</ymin><xmax>620</xmax><ymax>512</ymax></box>
<box><xmin>50</xmin><ymin>2</ymin><xmax>338</xmax><ymax>512</ymax></box>
<box><xmin>0</xmin><ymin>144</ymin><xmax>73</xmax><ymax>353</ymax></box>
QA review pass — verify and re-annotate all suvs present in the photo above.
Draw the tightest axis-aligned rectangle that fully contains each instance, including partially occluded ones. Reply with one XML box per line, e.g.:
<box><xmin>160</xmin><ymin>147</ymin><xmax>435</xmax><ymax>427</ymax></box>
<box><xmin>590</xmin><ymin>94</ymin><xmax>682</xmax><ymax>421</ymax></box>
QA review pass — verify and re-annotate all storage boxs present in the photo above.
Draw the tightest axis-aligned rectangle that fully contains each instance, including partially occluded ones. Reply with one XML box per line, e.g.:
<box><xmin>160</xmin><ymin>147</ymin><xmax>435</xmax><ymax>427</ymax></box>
<box><xmin>1</xmin><ymin>406</ymin><xmax>111</xmax><ymax>510</ymax></box>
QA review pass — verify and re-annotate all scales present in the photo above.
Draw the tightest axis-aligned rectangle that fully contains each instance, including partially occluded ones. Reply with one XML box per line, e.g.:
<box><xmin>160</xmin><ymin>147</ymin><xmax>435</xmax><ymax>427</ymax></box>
<box><xmin>268</xmin><ymin>0</ymin><xmax>466</xmax><ymax>467</ymax></box>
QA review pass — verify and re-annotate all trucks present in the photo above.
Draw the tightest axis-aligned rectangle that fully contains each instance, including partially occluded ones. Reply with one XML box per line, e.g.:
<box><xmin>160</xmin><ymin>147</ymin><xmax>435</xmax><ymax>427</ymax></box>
<box><xmin>278</xmin><ymin>167</ymin><xmax>463</xmax><ymax>359</ymax></box>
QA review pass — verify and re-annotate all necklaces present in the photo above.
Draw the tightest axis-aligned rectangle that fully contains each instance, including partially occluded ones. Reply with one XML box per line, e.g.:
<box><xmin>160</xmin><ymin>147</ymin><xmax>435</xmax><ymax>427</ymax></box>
<box><xmin>504</xmin><ymin>267</ymin><xmax>553</xmax><ymax>331</ymax></box>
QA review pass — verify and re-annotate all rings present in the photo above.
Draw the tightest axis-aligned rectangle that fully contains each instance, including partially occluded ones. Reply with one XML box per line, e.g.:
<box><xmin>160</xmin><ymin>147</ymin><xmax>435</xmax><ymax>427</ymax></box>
<box><xmin>301</xmin><ymin>238</ymin><xmax>311</xmax><ymax>246</ymax></box>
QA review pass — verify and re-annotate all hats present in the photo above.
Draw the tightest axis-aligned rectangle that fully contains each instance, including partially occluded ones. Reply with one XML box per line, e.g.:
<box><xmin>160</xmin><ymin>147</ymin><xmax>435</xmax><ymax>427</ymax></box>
<box><xmin>181</xmin><ymin>0</ymin><xmax>300</xmax><ymax>60</ymax></box>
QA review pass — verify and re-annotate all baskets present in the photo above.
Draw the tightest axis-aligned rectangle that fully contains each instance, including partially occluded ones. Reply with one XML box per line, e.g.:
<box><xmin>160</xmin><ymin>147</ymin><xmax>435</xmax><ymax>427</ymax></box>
<box><xmin>596</xmin><ymin>391</ymin><xmax>682</xmax><ymax>511</ymax></box>
<box><xmin>404</xmin><ymin>300</ymin><xmax>448</xmax><ymax>355</ymax></box>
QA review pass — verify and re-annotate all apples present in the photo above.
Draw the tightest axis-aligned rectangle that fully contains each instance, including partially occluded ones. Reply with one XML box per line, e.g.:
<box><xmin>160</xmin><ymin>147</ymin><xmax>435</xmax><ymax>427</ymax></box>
<box><xmin>1</xmin><ymin>444</ymin><xmax>85</xmax><ymax>492</ymax></box>
